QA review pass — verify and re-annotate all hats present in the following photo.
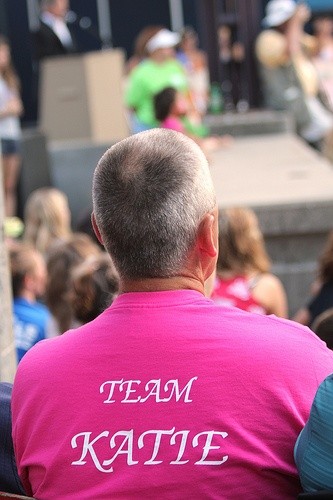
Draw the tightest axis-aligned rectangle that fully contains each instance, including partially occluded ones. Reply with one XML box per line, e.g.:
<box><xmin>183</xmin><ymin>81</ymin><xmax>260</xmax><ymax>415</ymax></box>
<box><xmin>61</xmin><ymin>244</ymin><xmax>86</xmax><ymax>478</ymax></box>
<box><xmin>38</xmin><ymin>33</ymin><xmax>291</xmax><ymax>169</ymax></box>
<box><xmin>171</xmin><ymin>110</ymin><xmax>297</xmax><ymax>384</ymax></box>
<box><xmin>262</xmin><ymin>1</ymin><xmax>296</xmax><ymax>26</ymax></box>
<box><xmin>145</xmin><ymin>28</ymin><xmax>180</xmax><ymax>55</ymax></box>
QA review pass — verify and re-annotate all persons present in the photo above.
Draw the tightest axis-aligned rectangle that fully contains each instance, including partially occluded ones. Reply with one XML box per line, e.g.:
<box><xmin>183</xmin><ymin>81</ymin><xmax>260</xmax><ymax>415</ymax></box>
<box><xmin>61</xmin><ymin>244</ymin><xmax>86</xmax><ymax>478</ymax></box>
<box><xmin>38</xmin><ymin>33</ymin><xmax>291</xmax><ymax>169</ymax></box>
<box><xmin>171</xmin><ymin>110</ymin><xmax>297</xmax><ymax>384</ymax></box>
<box><xmin>294</xmin><ymin>374</ymin><xmax>333</xmax><ymax>493</ymax></box>
<box><xmin>11</xmin><ymin>127</ymin><xmax>333</xmax><ymax>500</ymax></box>
<box><xmin>0</xmin><ymin>0</ymin><xmax>333</xmax><ymax>363</ymax></box>
